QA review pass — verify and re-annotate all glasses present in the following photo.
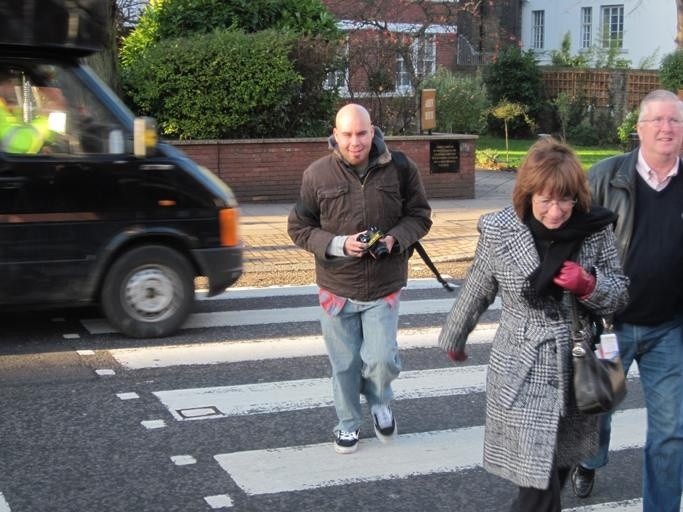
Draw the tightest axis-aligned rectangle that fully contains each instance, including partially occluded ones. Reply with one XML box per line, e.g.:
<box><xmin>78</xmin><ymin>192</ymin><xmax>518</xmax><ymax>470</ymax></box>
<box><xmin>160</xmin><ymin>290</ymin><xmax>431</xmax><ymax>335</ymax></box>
<box><xmin>639</xmin><ymin>115</ymin><xmax>681</xmax><ymax>129</ymax></box>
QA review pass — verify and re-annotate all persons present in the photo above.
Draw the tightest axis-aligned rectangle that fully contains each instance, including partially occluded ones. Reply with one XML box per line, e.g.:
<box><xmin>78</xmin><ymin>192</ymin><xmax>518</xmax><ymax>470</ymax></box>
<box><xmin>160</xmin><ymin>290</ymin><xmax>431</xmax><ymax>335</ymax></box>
<box><xmin>572</xmin><ymin>89</ymin><xmax>682</xmax><ymax>512</ymax></box>
<box><xmin>287</xmin><ymin>100</ymin><xmax>435</xmax><ymax>454</ymax></box>
<box><xmin>438</xmin><ymin>132</ymin><xmax>631</xmax><ymax>512</ymax></box>
<box><xmin>0</xmin><ymin>72</ymin><xmax>53</xmax><ymax>155</ymax></box>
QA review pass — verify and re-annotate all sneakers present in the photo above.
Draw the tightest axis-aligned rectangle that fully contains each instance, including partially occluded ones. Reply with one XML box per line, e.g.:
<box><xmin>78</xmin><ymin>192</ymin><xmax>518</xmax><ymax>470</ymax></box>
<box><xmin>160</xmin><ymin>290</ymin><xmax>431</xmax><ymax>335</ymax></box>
<box><xmin>371</xmin><ymin>403</ymin><xmax>398</xmax><ymax>442</ymax></box>
<box><xmin>332</xmin><ymin>427</ymin><xmax>361</xmax><ymax>453</ymax></box>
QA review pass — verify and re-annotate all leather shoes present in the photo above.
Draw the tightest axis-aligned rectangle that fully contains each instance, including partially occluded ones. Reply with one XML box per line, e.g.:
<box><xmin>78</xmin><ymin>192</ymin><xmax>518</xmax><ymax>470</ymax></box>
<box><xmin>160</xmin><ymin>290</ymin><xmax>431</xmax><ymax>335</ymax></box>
<box><xmin>571</xmin><ymin>464</ymin><xmax>595</xmax><ymax>497</ymax></box>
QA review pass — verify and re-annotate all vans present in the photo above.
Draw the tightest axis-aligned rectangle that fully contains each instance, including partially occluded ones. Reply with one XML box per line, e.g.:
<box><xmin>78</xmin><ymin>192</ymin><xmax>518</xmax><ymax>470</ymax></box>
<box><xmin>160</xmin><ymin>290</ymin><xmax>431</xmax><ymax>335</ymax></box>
<box><xmin>1</xmin><ymin>0</ymin><xmax>244</xmax><ymax>338</ymax></box>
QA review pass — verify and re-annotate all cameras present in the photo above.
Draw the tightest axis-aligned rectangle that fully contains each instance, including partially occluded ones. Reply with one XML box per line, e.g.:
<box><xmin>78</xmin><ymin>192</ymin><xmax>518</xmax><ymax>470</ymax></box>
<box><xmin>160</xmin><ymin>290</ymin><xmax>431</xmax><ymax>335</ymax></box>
<box><xmin>359</xmin><ymin>224</ymin><xmax>391</xmax><ymax>262</ymax></box>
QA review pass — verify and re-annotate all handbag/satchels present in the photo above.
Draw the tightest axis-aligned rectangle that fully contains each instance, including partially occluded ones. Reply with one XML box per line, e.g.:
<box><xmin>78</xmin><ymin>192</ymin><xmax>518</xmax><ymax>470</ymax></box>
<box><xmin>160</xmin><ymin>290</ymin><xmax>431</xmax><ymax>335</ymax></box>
<box><xmin>569</xmin><ymin>330</ymin><xmax>626</xmax><ymax>415</ymax></box>
